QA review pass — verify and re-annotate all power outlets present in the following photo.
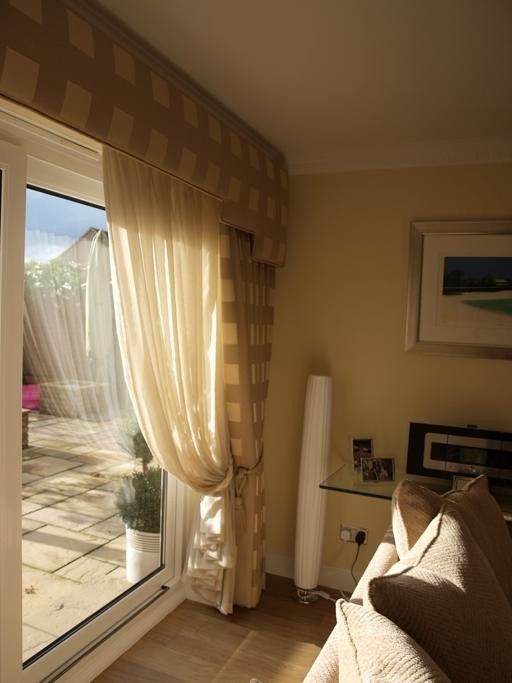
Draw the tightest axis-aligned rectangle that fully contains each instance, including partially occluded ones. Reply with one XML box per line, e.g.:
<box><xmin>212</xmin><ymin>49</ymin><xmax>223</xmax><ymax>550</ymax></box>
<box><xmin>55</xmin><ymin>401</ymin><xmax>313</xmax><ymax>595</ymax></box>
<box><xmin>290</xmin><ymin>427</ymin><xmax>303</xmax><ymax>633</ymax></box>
<box><xmin>339</xmin><ymin>522</ymin><xmax>368</xmax><ymax>545</ymax></box>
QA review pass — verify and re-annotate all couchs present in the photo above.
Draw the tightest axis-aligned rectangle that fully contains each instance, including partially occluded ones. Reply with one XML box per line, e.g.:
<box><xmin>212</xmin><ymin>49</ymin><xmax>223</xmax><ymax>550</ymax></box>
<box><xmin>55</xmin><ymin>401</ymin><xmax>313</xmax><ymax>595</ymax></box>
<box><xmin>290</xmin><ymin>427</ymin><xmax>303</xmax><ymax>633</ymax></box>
<box><xmin>302</xmin><ymin>512</ymin><xmax>512</xmax><ymax>683</ymax></box>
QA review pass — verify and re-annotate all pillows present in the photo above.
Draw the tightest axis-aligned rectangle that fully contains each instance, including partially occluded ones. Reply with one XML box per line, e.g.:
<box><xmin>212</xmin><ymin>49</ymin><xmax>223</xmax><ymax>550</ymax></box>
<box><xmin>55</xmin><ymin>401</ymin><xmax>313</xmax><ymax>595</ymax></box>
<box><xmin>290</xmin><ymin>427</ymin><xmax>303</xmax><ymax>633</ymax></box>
<box><xmin>335</xmin><ymin>474</ymin><xmax>512</xmax><ymax>683</ymax></box>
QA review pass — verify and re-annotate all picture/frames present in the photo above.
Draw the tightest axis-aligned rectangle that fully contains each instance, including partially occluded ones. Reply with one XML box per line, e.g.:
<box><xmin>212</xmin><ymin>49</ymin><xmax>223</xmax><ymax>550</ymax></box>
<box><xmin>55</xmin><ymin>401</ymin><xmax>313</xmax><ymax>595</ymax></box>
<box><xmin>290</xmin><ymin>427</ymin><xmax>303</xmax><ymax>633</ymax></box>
<box><xmin>360</xmin><ymin>457</ymin><xmax>395</xmax><ymax>485</ymax></box>
<box><xmin>404</xmin><ymin>218</ymin><xmax>512</xmax><ymax>360</ymax></box>
<box><xmin>351</xmin><ymin>436</ymin><xmax>375</xmax><ymax>473</ymax></box>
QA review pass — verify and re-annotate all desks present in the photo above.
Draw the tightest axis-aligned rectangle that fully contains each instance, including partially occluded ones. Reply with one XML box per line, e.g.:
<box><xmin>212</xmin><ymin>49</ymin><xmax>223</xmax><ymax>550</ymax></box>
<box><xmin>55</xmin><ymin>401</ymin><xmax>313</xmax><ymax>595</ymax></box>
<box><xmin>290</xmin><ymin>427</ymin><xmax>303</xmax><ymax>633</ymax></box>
<box><xmin>319</xmin><ymin>464</ymin><xmax>452</xmax><ymax>503</ymax></box>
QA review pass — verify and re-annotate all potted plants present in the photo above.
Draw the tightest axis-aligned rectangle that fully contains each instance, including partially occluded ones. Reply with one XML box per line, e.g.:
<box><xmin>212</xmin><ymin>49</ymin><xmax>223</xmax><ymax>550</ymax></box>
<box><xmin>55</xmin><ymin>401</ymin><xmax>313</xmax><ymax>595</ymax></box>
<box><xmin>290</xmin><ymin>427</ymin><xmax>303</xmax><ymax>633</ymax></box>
<box><xmin>119</xmin><ymin>431</ymin><xmax>152</xmax><ymax>504</ymax></box>
<box><xmin>111</xmin><ymin>466</ymin><xmax>163</xmax><ymax>584</ymax></box>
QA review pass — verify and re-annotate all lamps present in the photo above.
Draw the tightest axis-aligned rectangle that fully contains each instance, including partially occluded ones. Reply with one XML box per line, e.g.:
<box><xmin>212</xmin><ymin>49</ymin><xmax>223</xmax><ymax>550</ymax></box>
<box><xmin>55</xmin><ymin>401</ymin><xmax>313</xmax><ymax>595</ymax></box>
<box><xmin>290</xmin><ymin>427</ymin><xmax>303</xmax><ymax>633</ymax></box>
<box><xmin>291</xmin><ymin>374</ymin><xmax>333</xmax><ymax>605</ymax></box>
